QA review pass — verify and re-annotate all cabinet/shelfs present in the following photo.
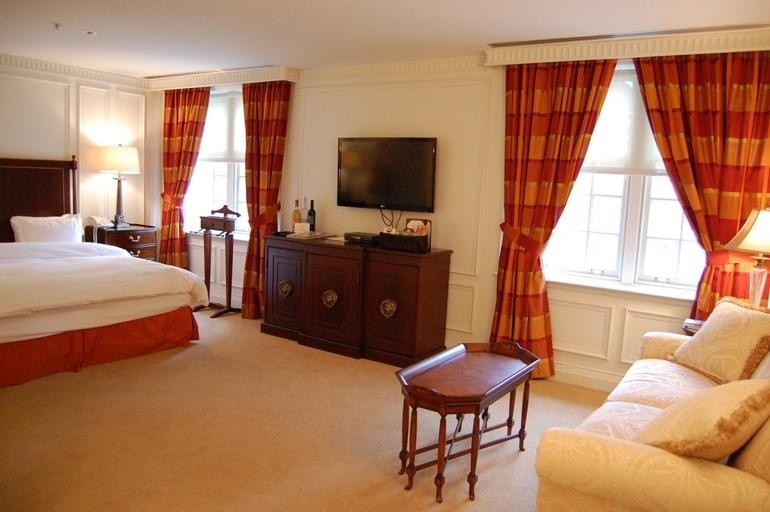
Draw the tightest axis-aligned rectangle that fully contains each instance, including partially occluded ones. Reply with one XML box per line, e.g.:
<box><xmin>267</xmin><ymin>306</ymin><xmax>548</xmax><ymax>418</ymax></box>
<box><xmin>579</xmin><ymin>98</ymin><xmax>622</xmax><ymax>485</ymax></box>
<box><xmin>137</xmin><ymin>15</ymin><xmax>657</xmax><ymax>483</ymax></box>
<box><xmin>261</xmin><ymin>231</ymin><xmax>453</xmax><ymax>368</ymax></box>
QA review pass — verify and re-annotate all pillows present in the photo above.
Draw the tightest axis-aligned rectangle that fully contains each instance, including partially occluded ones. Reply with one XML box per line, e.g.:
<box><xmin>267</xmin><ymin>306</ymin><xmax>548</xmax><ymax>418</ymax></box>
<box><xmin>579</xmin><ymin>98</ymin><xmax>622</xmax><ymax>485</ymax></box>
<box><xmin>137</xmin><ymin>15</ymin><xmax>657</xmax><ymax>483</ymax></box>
<box><xmin>670</xmin><ymin>295</ymin><xmax>770</xmax><ymax>385</ymax></box>
<box><xmin>632</xmin><ymin>379</ymin><xmax>770</xmax><ymax>462</ymax></box>
<box><xmin>9</xmin><ymin>213</ymin><xmax>85</xmax><ymax>243</ymax></box>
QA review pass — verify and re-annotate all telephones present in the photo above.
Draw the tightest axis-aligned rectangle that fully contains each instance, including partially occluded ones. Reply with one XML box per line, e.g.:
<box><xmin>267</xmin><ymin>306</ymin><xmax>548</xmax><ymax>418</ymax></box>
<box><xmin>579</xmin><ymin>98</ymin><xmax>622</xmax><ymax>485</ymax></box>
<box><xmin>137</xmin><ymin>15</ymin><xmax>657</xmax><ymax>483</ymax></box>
<box><xmin>87</xmin><ymin>216</ymin><xmax>114</xmax><ymax>243</ymax></box>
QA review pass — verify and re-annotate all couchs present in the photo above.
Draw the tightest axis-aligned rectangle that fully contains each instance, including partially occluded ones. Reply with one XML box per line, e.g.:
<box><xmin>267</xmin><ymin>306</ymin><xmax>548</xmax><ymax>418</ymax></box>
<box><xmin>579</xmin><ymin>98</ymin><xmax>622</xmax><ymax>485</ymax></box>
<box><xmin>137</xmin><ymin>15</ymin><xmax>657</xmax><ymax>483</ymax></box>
<box><xmin>536</xmin><ymin>332</ymin><xmax>770</xmax><ymax>512</ymax></box>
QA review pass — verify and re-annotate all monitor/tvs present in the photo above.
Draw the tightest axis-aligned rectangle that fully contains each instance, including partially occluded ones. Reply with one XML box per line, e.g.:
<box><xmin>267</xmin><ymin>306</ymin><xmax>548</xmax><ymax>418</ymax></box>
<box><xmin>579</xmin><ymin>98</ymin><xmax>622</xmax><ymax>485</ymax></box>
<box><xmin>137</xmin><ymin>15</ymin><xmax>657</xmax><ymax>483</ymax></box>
<box><xmin>337</xmin><ymin>137</ymin><xmax>438</xmax><ymax>214</ymax></box>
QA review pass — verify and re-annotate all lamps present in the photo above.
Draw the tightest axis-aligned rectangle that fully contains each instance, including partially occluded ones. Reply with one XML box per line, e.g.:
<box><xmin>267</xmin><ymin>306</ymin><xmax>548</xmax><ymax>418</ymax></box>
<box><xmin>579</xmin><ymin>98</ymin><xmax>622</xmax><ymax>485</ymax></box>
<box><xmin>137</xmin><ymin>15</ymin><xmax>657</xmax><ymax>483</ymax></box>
<box><xmin>96</xmin><ymin>143</ymin><xmax>141</xmax><ymax>228</ymax></box>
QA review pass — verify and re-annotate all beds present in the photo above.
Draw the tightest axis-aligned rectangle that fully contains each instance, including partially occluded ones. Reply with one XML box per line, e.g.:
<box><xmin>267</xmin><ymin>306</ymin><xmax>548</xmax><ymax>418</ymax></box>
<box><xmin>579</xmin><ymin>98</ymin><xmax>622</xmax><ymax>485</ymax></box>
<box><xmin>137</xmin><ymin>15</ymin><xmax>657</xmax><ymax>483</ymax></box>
<box><xmin>724</xmin><ymin>207</ymin><xmax>770</xmax><ymax>309</ymax></box>
<box><xmin>0</xmin><ymin>154</ymin><xmax>209</xmax><ymax>388</ymax></box>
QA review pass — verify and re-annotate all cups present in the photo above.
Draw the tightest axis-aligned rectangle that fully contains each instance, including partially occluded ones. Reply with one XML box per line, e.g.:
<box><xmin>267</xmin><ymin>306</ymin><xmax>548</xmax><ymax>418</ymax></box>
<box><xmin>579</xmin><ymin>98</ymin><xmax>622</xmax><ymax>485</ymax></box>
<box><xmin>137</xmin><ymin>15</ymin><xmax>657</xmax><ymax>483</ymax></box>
<box><xmin>295</xmin><ymin>224</ymin><xmax>311</xmax><ymax>233</ymax></box>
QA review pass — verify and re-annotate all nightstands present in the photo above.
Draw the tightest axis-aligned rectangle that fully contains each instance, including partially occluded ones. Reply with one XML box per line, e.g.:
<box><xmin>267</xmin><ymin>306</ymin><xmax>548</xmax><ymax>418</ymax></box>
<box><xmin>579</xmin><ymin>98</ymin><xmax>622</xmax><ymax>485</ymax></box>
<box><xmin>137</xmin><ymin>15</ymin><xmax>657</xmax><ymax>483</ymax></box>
<box><xmin>84</xmin><ymin>221</ymin><xmax>158</xmax><ymax>263</ymax></box>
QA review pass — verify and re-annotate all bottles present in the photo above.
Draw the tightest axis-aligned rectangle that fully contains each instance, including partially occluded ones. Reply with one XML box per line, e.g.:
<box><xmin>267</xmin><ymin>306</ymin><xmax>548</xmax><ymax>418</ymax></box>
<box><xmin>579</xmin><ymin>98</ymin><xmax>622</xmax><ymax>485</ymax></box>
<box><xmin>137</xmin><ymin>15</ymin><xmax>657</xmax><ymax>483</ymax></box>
<box><xmin>390</xmin><ymin>224</ymin><xmax>399</xmax><ymax>235</ymax></box>
<box><xmin>300</xmin><ymin>198</ymin><xmax>308</xmax><ymax>223</ymax></box>
<box><xmin>308</xmin><ymin>200</ymin><xmax>316</xmax><ymax>231</ymax></box>
<box><xmin>291</xmin><ymin>199</ymin><xmax>301</xmax><ymax>234</ymax></box>
